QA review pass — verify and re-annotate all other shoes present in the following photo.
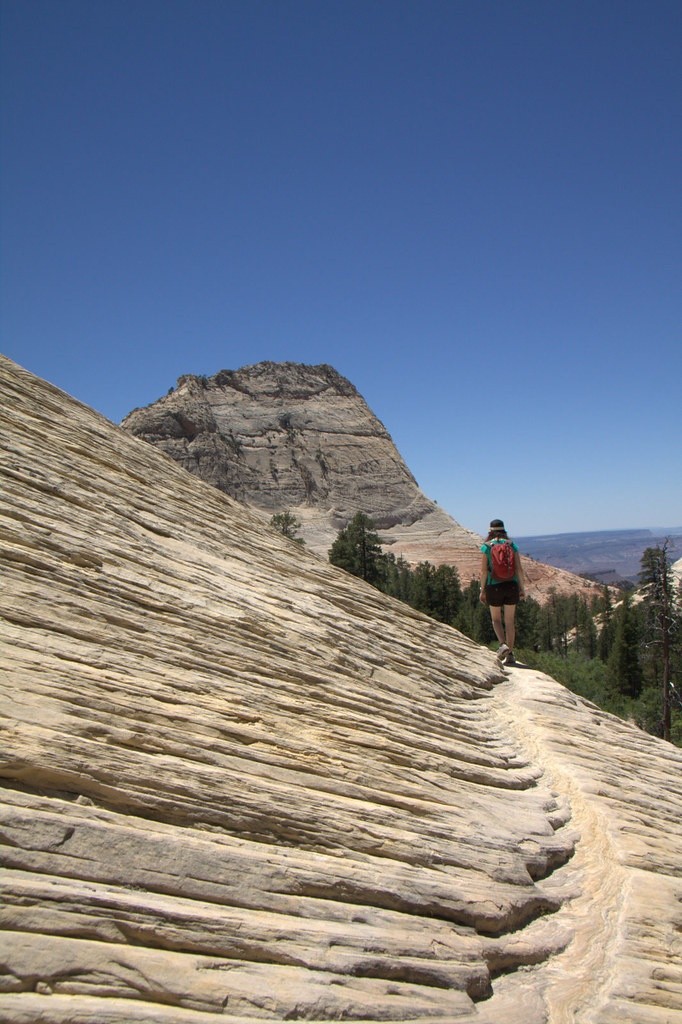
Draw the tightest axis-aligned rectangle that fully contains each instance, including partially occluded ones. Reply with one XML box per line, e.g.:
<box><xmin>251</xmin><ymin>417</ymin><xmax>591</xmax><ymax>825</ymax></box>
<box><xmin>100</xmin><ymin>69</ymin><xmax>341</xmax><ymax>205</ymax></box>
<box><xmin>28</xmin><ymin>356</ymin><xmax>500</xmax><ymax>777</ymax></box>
<box><xmin>497</xmin><ymin>645</ymin><xmax>510</xmax><ymax>661</ymax></box>
<box><xmin>504</xmin><ymin>655</ymin><xmax>515</xmax><ymax>665</ymax></box>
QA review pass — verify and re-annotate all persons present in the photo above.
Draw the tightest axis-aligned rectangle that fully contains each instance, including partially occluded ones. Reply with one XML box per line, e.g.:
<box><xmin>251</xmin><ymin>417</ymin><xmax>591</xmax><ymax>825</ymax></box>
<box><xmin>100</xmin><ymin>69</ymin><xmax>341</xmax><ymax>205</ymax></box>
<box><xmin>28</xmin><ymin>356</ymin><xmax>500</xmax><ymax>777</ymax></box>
<box><xmin>479</xmin><ymin>519</ymin><xmax>525</xmax><ymax>664</ymax></box>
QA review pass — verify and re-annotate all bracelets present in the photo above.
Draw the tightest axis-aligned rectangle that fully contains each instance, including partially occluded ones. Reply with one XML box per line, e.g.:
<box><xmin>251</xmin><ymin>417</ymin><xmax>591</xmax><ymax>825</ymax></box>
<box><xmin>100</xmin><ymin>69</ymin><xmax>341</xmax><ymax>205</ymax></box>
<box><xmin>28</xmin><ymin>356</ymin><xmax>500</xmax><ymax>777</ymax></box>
<box><xmin>480</xmin><ymin>588</ymin><xmax>485</xmax><ymax>591</ymax></box>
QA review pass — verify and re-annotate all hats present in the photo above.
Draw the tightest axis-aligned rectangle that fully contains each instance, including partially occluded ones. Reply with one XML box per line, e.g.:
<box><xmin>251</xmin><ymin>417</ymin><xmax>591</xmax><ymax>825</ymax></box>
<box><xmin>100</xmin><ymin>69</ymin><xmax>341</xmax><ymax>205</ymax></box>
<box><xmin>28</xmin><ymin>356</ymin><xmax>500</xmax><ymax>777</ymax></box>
<box><xmin>489</xmin><ymin>519</ymin><xmax>504</xmax><ymax>530</ymax></box>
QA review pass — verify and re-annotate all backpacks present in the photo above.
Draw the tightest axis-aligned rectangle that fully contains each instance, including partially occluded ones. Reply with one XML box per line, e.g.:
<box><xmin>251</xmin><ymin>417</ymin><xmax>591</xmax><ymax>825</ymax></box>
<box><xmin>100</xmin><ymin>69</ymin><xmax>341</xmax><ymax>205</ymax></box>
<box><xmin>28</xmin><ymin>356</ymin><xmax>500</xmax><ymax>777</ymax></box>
<box><xmin>484</xmin><ymin>540</ymin><xmax>516</xmax><ymax>581</ymax></box>
